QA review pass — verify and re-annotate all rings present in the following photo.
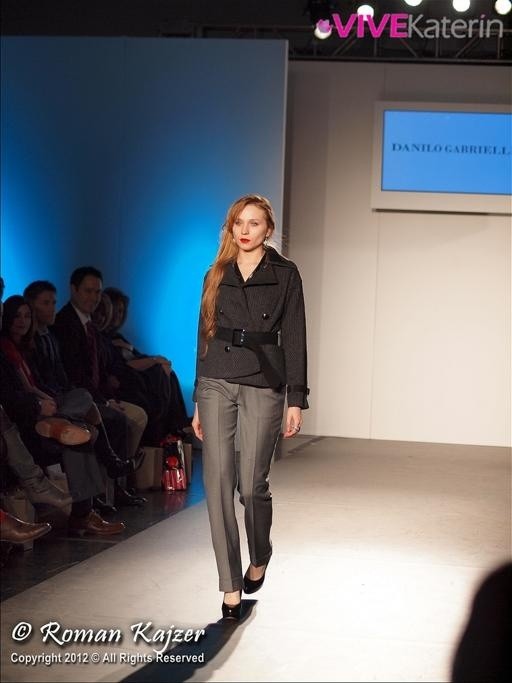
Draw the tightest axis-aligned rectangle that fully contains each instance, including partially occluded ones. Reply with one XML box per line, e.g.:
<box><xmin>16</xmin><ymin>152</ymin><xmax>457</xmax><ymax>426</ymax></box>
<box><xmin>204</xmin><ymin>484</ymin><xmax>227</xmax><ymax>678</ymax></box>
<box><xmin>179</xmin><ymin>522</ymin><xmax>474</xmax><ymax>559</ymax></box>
<box><xmin>294</xmin><ymin>426</ymin><xmax>299</xmax><ymax>430</ymax></box>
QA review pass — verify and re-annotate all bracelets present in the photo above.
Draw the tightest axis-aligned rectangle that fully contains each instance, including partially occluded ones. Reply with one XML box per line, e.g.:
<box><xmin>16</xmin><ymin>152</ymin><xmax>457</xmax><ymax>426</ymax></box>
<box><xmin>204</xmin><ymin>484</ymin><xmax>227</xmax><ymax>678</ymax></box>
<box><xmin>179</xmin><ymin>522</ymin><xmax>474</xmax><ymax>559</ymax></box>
<box><xmin>130</xmin><ymin>343</ymin><xmax>134</xmax><ymax>351</ymax></box>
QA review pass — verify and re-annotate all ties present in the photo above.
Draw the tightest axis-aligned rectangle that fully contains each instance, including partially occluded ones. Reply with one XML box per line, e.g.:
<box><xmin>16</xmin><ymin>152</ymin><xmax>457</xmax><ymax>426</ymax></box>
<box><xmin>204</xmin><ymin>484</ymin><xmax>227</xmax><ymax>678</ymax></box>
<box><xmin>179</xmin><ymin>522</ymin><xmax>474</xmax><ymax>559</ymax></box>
<box><xmin>84</xmin><ymin>322</ymin><xmax>100</xmax><ymax>389</ymax></box>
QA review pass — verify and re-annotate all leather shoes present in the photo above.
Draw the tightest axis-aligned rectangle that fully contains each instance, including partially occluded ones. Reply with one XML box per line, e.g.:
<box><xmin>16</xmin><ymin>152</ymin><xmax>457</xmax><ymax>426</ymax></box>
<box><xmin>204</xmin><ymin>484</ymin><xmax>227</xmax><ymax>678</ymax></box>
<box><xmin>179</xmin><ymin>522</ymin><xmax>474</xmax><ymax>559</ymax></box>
<box><xmin>119</xmin><ymin>490</ymin><xmax>150</xmax><ymax>508</ymax></box>
<box><xmin>109</xmin><ymin>452</ymin><xmax>146</xmax><ymax>479</ymax></box>
<box><xmin>242</xmin><ymin>571</ymin><xmax>266</xmax><ymax>594</ymax></box>
<box><xmin>221</xmin><ymin>590</ymin><xmax>242</xmax><ymax>623</ymax></box>
<box><xmin>70</xmin><ymin>510</ymin><xmax>127</xmax><ymax>537</ymax></box>
<box><xmin>0</xmin><ymin>512</ymin><xmax>52</xmax><ymax>543</ymax></box>
<box><xmin>37</xmin><ymin>417</ymin><xmax>89</xmax><ymax>446</ymax></box>
<box><xmin>15</xmin><ymin>480</ymin><xmax>76</xmax><ymax>508</ymax></box>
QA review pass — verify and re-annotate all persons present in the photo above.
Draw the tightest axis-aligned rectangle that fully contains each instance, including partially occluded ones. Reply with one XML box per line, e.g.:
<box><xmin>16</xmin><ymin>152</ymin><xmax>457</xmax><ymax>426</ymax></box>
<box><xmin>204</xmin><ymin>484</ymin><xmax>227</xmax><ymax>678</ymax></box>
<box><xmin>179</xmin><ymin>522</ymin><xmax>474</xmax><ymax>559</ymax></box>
<box><xmin>4</xmin><ymin>295</ymin><xmax>145</xmax><ymax>506</ymax></box>
<box><xmin>53</xmin><ymin>265</ymin><xmax>149</xmax><ymax>507</ymax></box>
<box><xmin>106</xmin><ymin>286</ymin><xmax>193</xmax><ymax>442</ymax></box>
<box><xmin>0</xmin><ymin>508</ymin><xmax>51</xmax><ymax>543</ymax></box>
<box><xmin>23</xmin><ymin>280</ymin><xmax>117</xmax><ymax>514</ymax></box>
<box><xmin>1</xmin><ymin>277</ymin><xmax>75</xmax><ymax>506</ymax></box>
<box><xmin>94</xmin><ymin>292</ymin><xmax>158</xmax><ymax>444</ymax></box>
<box><xmin>189</xmin><ymin>195</ymin><xmax>309</xmax><ymax>617</ymax></box>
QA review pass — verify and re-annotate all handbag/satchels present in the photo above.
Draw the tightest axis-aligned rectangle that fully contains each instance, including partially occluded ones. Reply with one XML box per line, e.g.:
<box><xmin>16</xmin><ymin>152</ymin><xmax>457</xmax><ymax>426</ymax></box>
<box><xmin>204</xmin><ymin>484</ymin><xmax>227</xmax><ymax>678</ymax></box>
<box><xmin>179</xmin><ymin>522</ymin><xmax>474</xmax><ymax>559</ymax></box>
<box><xmin>133</xmin><ymin>446</ymin><xmax>163</xmax><ymax>491</ymax></box>
<box><xmin>158</xmin><ymin>434</ymin><xmax>186</xmax><ymax>469</ymax></box>
<box><xmin>182</xmin><ymin>441</ymin><xmax>194</xmax><ymax>485</ymax></box>
<box><xmin>161</xmin><ymin>468</ymin><xmax>186</xmax><ymax>493</ymax></box>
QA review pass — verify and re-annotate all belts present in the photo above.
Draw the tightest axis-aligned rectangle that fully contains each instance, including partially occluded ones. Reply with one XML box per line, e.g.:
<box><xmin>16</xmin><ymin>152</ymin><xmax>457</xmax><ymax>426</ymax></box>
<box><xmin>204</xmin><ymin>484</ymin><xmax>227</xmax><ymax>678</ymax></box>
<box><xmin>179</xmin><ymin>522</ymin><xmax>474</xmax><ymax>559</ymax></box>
<box><xmin>210</xmin><ymin>327</ymin><xmax>289</xmax><ymax>391</ymax></box>
